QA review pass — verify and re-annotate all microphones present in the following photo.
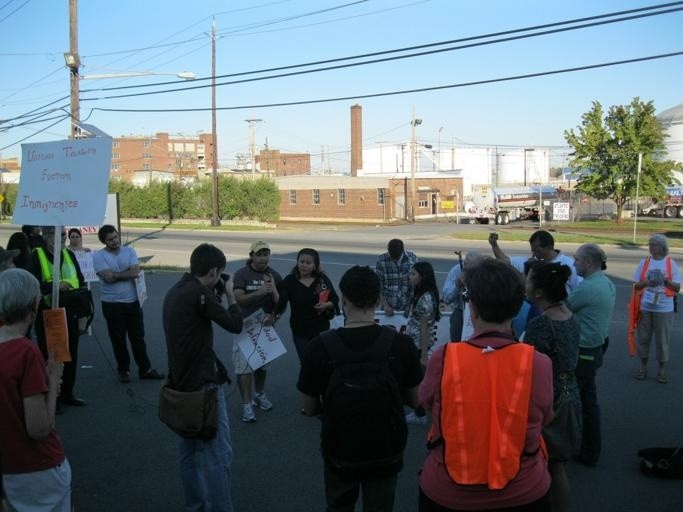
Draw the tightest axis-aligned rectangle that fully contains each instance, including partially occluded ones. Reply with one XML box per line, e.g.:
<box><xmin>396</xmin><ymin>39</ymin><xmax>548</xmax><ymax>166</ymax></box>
<box><xmin>264</xmin><ymin>263</ymin><xmax>272</xmax><ymax>282</ymax></box>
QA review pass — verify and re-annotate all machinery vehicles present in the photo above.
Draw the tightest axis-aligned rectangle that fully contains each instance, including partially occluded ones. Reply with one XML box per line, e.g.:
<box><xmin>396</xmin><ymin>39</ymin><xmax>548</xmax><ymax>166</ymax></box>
<box><xmin>462</xmin><ymin>184</ymin><xmax>566</xmax><ymax>225</ymax></box>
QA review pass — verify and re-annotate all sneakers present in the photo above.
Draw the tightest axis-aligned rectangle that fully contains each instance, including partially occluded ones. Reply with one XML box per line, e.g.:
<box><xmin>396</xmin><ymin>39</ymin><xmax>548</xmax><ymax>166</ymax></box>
<box><xmin>406</xmin><ymin>411</ymin><xmax>427</xmax><ymax>426</ymax></box>
<box><xmin>253</xmin><ymin>391</ymin><xmax>273</xmax><ymax>411</ymax></box>
<box><xmin>139</xmin><ymin>369</ymin><xmax>164</xmax><ymax>379</ymax></box>
<box><xmin>242</xmin><ymin>403</ymin><xmax>256</xmax><ymax>422</ymax></box>
<box><xmin>118</xmin><ymin>371</ymin><xmax>131</xmax><ymax>382</ymax></box>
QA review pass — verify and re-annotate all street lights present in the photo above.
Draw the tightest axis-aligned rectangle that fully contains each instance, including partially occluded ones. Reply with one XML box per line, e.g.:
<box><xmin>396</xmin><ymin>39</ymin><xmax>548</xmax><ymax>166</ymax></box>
<box><xmin>524</xmin><ymin>148</ymin><xmax>536</xmax><ymax>185</ymax></box>
<box><xmin>62</xmin><ymin>0</ymin><xmax>197</xmax><ymax>138</ymax></box>
<box><xmin>410</xmin><ymin>104</ymin><xmax>432</xmax><ymax>224</ymax></box>
<box><xmin>437</xmin><ymin>125</ymin><xmax>444</xmax><ymax>173</ymax></box>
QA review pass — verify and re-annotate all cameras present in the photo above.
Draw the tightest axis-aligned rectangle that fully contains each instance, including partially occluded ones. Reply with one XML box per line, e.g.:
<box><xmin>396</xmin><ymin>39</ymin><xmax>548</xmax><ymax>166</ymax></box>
<box><xmin>214</xmin><ymin>273</ymin><xmax>230</xmax><ymax>296</ymax></box>
<box><xmin>491</xmin><ymin>233</ymin><xmax>498</xmax><ymax>241</ymax></box>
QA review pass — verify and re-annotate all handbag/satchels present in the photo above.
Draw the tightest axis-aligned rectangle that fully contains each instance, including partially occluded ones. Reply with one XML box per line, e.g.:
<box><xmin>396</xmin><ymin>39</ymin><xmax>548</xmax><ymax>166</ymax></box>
<box><xmin>159</xmin><ymin>376</ymin><xmax>218</xmax><ymax>442</ymax></box>
<box><xmin>59</xmin><ymin>287</ymin><xmax>91</xmax><ymax>322</ymax></box>
<box><xmin>543</xmin><ymin>391</ymin><xmax>579</xmax><ymax>462</ymax></box>
<box><xmin>310</xmin><ymin>289</ymin><xmax>334</xmax><ymax>322</ymax></box>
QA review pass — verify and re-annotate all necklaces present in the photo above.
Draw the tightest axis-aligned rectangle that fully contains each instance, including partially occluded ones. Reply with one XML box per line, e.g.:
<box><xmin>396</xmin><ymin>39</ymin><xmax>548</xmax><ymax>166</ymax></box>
<box><xmin>539</xmin><ymin>302</ymin><xmax>564</xmax><ymax>312</ymax></box>
<box><xmin>345</xmin><ymin>320</ymin><xmax>375</xmax><ymax>324</ymax></box>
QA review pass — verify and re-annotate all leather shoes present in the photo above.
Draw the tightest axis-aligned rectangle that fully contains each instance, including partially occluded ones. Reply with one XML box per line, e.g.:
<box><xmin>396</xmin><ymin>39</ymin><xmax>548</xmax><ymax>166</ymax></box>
<box><xmin>56</xmin><ymin>396</ymin><xmax>85</xmax><ymax>415</ymax></box>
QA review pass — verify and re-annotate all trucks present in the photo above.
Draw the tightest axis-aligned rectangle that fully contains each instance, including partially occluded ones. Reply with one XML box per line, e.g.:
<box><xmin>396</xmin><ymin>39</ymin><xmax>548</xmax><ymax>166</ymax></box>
<box><xmin>646</xmin><ymin>187</ymin><xmax>682</xmax><ymax>219</ymax></box>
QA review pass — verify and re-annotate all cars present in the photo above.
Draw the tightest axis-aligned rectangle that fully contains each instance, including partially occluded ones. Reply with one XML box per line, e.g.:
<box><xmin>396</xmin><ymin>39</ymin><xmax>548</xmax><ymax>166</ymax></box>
<box><xmin>642</xmin><ymin>201</ymin><xmax>663</xmax><ymax>216</ymax></box>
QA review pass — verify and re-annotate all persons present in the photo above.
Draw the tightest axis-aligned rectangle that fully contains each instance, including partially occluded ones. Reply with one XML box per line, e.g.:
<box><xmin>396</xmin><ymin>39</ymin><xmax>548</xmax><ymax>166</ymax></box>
<box><xmin>0</xmin><ymin>269</ymin><xmax>71</xmax><ymax>511</ymax></box>
<box><xmin>417</xmin><ymin>258</ymin><xmax>556</xmax><ymax>512</ymax></box>
<box><xmin>632</xmin><ymin>233</ymin><xmax>680</xmax><ymax>383</ymax></box>
<box><xmin>524</xmin><ymin>261</ymin><xmax>584</xmax><ymax>511</ymax></box>
<box><xmin>296</xmin><ymin>263</ymin><xmax>424</xmax><ymax>511</ymax></box>
<box><xmin>375</xmin><ymin>230</ymin><xmax>618</xmax><ymax>467</ymax></box>
<box><xmin>262</xmin><ymin>247</ymin><xmax>341</xmax><ymax>414</ymax></box>
<box><xmin>162</xmin><ymin>243</ymin><xmax>243</xmax><ymax>511</ymax></box>
<box><xmin>1</xmin><ymin>226</ymin><xmax>166</xmax><ymax>414</ymax></box>
<box><xmin>233</xmin><ymin>239</ymin><xmax>288</xmax><ymax>422</ymax></box>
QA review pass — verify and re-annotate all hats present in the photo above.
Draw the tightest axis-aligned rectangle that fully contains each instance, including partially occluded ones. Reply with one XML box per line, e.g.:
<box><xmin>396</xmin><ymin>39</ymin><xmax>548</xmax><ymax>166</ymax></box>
<box><xmin>250</xmin><ymin>241</ymin><xmax>271</xmax><ymax>253</ymax></box>
<box><xmin>0</xmin><ymin>246</ymin><xmax>20</xmax><ymax>265</ymax></box>
